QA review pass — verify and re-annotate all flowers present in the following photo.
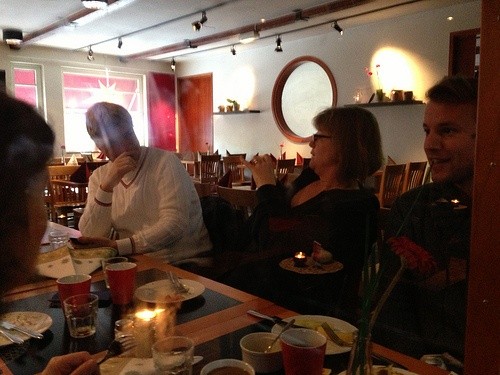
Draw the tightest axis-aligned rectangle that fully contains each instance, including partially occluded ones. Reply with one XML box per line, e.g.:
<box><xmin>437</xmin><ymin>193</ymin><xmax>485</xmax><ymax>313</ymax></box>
<box><xmin>363</xmin><ymin>63</ymin><xmax>383</xmax><ymax>89</ymax></box>
<box><xmin>348</xmin><ymin>235</ymin><xmax>434</xmax><ymax>375</ymax></box>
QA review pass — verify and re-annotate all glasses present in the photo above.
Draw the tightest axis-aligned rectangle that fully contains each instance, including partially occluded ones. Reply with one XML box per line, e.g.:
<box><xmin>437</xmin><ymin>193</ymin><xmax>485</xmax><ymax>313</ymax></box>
<box><xmin>310</xmin><ymin>134</ymin><xmax>329</xmax><ymax>142</ymax></box>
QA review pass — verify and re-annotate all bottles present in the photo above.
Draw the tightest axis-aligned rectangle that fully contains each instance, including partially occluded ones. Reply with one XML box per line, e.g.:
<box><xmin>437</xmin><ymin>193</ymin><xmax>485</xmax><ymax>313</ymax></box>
<box><xmin>114</xmin><ymin>306</ymin><xmax>175</xmax><ymax>357</ymax></box>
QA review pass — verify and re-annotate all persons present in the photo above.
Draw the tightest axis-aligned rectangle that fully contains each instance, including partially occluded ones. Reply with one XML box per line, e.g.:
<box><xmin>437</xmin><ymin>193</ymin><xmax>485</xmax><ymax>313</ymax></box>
<box><xmin>228</xmin><ymin>73</ymin><xmax>477</xmax><ymax>372</ymax></box>
<box><xmin>0</xmin><ymin>91</ymin><xmax>101</xmax><ymax>375</ymax></box>
<box><xmin>69</xmin><ymin>102</ymin><xmax>218</xmax><ymax>280</ymax></box>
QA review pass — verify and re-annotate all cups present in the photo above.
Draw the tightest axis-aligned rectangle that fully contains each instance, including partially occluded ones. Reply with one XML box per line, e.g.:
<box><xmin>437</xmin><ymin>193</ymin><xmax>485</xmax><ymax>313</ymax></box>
<box><xmin>240</xmin><ymin>331</ymin><xmax>286</xmax><ymax>375</ymax></box>
<box><xmin>101</xmin><ymin>256</ymin><xmax>128</xmax><ymax>288</ymax></box>
<box><xmin>280</xmin><ymin>329</ymin><xmax>327</xmax><ymax>375</ymax></box>
<box><xmin>294</xmin><ymin>251</ymin><xmax>307</xmax><ymax>266</ymax></box>
<box><xmin>150</xmin><ymin>336</ymin><xmax>194</xmax><ymax>375</ymax></box>
<box><xmin>50</xmin><ymin>229</ymin><xmax>70</xmax><ymax>251</ymax></box>
<box><xmin>56</xmin><ymin>273</ymin><xmax>92</xmax><ymax>320</ymax></box>
<box><xmin>200</xmin><ymin>358</ymin><xmax>256</xmax><ymax>375</ymax></box>
<box><xmin>219</xmin><ymin>105</ymin><xmax>240</xmax><ymax>112</ymax></box>
<box><xmin>62</xmin><ymin>294</ymin><xmax>98</xmax><ymax>337</ymax></box>
<box><xmin>105</xmin><ymin>262</ymin><xmax>138</xmax><ymax>305</ymax></box>
<box><xmin>352</xmin><ymin>89</ymin><xmax>413</xmax><ymax>103</ymax></box>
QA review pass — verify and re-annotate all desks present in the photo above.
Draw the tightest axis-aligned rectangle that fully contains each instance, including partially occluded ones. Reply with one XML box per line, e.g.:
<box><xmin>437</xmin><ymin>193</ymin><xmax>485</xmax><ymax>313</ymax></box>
<box><xmin>0</xmin><ymin>220</ymin><xmax>455</xmax><ymax>375</ymax></box>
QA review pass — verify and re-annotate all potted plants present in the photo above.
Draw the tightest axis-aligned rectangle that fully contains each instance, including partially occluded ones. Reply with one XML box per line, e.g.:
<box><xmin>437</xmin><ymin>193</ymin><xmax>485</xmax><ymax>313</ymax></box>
<box><xmin>226</xmin><ymin>99</ymin><xmax>239</xmax><ymax>111</ymax></box>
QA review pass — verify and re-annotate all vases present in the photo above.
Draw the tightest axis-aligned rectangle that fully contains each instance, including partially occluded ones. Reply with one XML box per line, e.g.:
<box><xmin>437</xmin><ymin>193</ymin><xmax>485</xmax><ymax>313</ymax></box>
<box><xmin>375</xmin><ymin>90</ymin><xmax>384</xmax><ymax>103</ymax></box>
<box><xmin>347</xmin><ymin>331</ymin><xmax>375</xmax><ymax>375</ymax></box>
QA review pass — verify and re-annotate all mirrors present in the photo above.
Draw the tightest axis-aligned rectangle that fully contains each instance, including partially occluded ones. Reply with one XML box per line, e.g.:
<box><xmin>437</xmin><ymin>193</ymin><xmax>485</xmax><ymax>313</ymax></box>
<box><xmin>272</xmin><ymin>55</ymin><xmax>338</xmax><ymax>143</ymax></box>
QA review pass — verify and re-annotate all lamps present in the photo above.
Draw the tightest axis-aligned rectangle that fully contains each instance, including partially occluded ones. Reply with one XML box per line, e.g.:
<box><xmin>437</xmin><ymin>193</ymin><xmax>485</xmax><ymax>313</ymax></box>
<box><xmin>192</xmin><ymin>10</ymin><xmax>208</xmax><ymax>32</ymax></box>
<box><xmin>330</xmin><ymin>21</ymin><xmax>344</xmax><ymax>36</ymax></box>
<box><xmin>275</xmin><ymin>34</ymin><xmax>283</xmax><ymax>53</ymax></box>
<box><xmin>87</xmin><ymin>47</ymin><xmax>95</xmax><ymax>62</ymax></box>
<box><xmin>170</xmin><ymin>57</ymin><xmax>177</xmax><ymax>73</ymax></box>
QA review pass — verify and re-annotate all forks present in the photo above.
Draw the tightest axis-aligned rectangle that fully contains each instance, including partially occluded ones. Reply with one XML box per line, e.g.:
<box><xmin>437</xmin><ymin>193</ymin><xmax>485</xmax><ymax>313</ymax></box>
<box><xmin>96</xmin><ymin>333</ymin><xmax>138</xmax><ymax>367</ymax></box>
<box><xmin>321</xmin><ymin>322</ymin><xmax>391</xmax><ymax>364</ymax></box>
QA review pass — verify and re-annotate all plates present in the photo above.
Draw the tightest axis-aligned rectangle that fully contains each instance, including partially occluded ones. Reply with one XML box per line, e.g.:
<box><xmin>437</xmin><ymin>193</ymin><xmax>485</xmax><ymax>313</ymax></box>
<box><xmin>135</xmin><ymin>279</ymin><xmax>204</xmax><ymax>304</ymax></box>
<box><xmin>338</xmin><ymin>364</ymin><xmax>418</xmax><ymax>375</ymax></box>
<box><xmin>0</xmin><ymin>311</ymin><xmax>52</xmax><ymax>348</ymax></box>
<box><xmin>271</xmin><ymin>315</ymin><xmax>359</xmax><ymax>355</ymax></box>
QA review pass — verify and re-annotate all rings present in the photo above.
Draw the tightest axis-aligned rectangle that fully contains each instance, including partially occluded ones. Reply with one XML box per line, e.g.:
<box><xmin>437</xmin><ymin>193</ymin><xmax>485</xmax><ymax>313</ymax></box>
<box><xmin>253</xmin><ymin>158</ymin><xmax>260</xmax><ymax>163</ymax></box>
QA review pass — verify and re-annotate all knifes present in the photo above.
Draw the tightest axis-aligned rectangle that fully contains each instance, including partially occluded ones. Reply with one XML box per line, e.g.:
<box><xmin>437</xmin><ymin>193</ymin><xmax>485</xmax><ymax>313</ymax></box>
<box><xmin>0</xmin><ymin>320</ymin><xmax>44</xmax><ymax>339</ymax></box>
<box><xmin>248</xmin><ymin>309</ymin><xmax>314</xmax><ymax>330</ymax></box>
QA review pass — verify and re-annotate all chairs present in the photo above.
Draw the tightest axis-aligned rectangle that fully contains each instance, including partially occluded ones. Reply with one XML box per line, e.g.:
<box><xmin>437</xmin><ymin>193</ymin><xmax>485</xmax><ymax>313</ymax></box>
<box><xmin>45</xmin><ymin>151</ymin><xmax>431</xmax><ymax>226</ymax></box>
